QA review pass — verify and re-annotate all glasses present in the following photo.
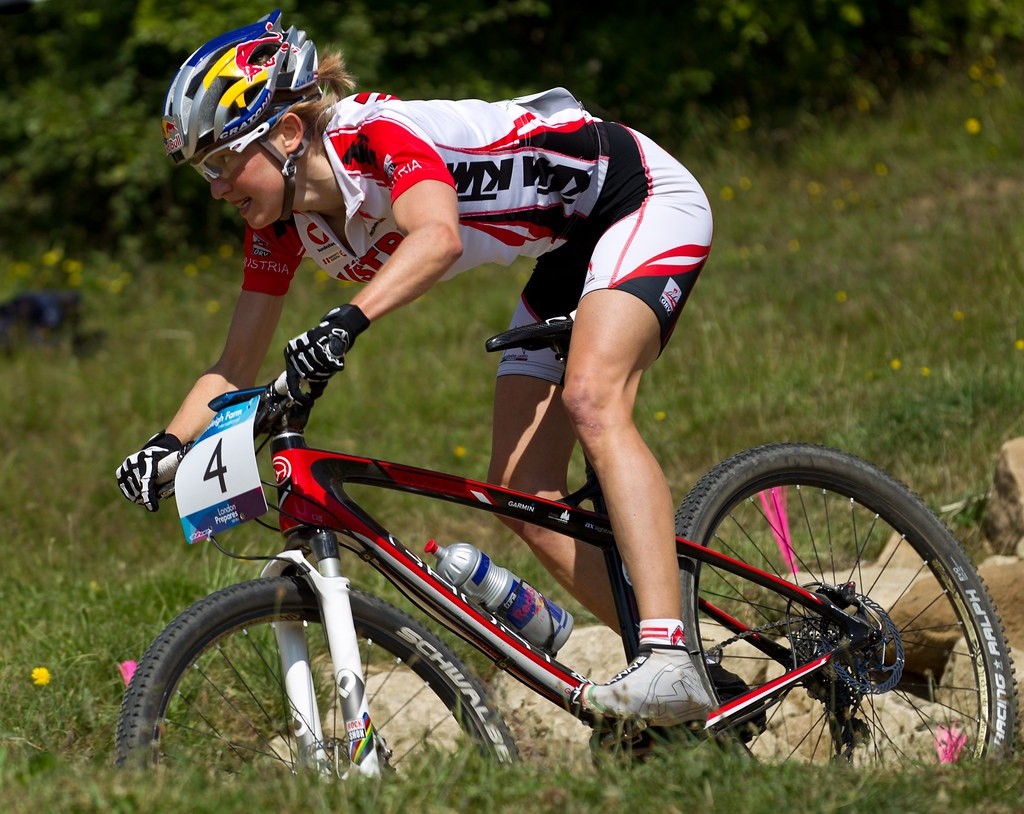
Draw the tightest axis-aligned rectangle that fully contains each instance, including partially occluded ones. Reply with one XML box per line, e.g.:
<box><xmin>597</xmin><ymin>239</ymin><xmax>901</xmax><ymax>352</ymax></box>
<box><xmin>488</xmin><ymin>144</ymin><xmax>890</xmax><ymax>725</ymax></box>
<box><xmin>191</xmin><ymin>93</ymin><xmax>322</xmax><ymax>183</ymax></box>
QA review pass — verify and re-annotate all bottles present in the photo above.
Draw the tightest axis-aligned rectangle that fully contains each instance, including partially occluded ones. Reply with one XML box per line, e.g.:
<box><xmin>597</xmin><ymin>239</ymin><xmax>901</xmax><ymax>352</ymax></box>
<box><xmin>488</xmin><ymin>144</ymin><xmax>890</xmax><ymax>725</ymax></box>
<box><xmin>424</xmin><ymin>537</ymin><xmax>574</xmax><ymax>654</ymax></box>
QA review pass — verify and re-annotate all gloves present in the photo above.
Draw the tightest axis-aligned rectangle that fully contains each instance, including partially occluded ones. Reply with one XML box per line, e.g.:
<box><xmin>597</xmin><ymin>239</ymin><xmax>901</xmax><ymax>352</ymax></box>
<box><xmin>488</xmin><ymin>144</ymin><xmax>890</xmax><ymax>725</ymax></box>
<box><xmin>113</xmin><ymin>430</ymin><xmax>184</xmax><ymax>513</ymax></box>
<box><xmin>283</xmin><ymin>304</ymin><xmax>372</xmax><ymax>408</ymax></box>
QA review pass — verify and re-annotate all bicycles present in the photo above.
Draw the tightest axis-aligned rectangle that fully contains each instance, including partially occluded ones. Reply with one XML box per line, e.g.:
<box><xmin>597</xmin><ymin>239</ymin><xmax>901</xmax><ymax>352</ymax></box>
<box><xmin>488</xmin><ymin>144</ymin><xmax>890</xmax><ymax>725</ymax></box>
<box><xmin>111</xmin><ymin>311</ymin><xmax>1020</xmax><ymax>814</ymax></box>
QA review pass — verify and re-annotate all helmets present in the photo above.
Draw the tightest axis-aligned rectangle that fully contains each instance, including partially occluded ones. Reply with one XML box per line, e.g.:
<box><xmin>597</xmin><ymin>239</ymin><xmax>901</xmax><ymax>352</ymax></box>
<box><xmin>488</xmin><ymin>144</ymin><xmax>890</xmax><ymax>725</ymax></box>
<box><xmin>162</xmin><ymin>10</ymin><xmax>319</xmax><ymax>167</ymax></box>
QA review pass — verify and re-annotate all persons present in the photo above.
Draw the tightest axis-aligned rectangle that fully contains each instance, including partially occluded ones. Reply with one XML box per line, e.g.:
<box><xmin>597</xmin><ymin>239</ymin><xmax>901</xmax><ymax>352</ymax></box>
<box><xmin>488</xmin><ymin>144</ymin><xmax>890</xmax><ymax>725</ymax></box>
<box><xmin>116</xmin><ymin>9</ymin><xmax>768</xmax><ymax>768</ymax></box>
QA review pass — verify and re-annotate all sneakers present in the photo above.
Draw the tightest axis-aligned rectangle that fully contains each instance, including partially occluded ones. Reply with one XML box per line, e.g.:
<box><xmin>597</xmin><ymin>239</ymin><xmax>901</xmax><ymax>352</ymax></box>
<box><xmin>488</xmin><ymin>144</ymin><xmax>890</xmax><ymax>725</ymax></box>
<box><xmin>582</xmin><ymin>642</ymin><xmax>721</xmax><ymax>728</ymax></box>
